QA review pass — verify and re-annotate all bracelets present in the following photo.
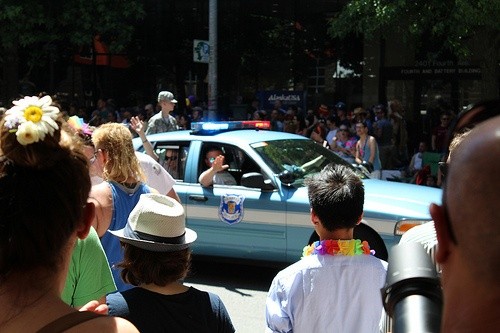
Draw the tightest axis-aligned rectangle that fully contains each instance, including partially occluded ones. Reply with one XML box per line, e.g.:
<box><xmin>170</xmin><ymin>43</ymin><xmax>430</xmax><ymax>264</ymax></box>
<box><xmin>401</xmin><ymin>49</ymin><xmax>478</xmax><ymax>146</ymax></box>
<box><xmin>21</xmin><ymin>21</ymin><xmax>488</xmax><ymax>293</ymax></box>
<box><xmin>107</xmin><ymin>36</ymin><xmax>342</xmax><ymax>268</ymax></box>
<box><xmin>142</xmin><ymin>139</ymin><xmax>148</xmax><ymax>143</ymax></box>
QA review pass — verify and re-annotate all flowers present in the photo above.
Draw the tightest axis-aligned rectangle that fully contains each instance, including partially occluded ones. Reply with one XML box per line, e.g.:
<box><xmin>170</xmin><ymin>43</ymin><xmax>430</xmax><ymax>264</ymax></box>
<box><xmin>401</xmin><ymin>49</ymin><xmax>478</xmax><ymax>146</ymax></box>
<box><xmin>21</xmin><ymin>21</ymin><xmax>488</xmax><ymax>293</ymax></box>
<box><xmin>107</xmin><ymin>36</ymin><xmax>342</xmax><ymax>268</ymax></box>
<box><xmin>4</xmin><ymin>95</ymin><xmax>60</xmax><ymax>145</ymax></box>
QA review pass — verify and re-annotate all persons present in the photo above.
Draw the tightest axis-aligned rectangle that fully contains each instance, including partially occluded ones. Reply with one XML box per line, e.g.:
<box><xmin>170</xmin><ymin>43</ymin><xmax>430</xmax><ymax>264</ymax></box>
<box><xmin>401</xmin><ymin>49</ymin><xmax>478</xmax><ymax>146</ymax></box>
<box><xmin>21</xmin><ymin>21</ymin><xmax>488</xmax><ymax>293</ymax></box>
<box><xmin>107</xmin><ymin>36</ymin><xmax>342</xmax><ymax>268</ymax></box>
<box><xmin>0</xmin><ymin>90</ymin><xmax>500</xmax><ymax>333</ymax></box>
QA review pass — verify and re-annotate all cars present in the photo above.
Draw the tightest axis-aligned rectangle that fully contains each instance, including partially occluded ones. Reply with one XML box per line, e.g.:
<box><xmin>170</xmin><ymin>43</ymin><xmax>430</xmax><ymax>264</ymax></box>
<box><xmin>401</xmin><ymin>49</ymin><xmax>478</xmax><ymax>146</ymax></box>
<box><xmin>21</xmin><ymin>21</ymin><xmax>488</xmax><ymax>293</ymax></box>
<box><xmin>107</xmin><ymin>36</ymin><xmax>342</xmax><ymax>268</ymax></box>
<box><xmin>132</xmin><ymin>120</ymin><xmax>462</xmax><ymax>288</ymax></box>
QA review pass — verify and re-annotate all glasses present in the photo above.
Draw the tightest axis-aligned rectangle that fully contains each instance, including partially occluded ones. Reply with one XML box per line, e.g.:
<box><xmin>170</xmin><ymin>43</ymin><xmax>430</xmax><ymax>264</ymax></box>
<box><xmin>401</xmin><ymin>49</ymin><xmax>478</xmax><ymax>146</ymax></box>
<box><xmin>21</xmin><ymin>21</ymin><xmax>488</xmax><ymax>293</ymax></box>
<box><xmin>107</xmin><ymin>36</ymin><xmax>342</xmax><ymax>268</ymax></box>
<box><xmin>207</xmin><ymin>157</ymin><xmax>215</xmax><ymax>163</ymax></box>
<box><xmin>165</xmin><ymin>155</ymin><xmax>177</xmax><ymax>161</ymax></box>
<box><xmin>339</xmin><ymin>129</ymin><xmax>348</xmax><ymax>132</ymax></box>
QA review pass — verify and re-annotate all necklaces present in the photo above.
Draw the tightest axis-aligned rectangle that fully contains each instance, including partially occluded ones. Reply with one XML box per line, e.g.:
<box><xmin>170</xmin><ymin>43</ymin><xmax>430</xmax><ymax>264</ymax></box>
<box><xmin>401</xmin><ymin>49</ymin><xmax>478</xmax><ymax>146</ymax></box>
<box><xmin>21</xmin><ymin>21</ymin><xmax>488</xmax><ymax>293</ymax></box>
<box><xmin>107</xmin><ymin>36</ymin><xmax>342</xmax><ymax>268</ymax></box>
<box><xmin>302</xmin><ymin>239</ymin><xmax>375</xmax><ymax>257</ymax></box>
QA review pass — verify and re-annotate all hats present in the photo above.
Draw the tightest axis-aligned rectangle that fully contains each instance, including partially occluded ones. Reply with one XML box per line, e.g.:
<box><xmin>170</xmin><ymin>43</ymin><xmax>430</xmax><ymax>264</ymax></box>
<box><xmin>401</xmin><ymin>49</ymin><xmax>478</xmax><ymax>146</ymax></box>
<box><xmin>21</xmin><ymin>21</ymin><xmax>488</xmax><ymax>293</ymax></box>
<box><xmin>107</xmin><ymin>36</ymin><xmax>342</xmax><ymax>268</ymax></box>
<box><xmin>158</xmin><ymin>91</ymin><xmax>178</xmax><ymax>103</ymax></box>
<box><xmin>106</xmin><ymin>193</ymin><xmax>197</xmax><ymax>250</ymax></box>
